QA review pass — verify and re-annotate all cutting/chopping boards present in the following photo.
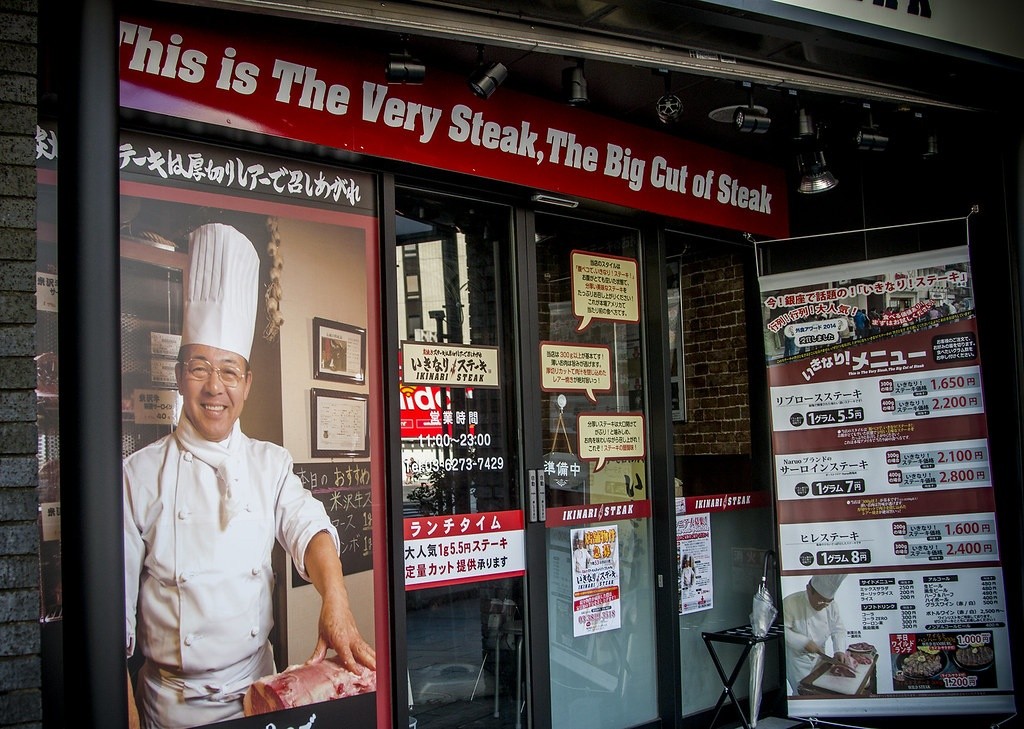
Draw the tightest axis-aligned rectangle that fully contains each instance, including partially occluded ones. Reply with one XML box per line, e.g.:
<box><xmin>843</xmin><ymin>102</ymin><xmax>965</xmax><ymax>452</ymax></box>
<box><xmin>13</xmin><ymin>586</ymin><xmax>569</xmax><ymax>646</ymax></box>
<box><xmin>811</xmin><ymin>662</ymin><xmax>871</xmax><ymax>695</ymax></box>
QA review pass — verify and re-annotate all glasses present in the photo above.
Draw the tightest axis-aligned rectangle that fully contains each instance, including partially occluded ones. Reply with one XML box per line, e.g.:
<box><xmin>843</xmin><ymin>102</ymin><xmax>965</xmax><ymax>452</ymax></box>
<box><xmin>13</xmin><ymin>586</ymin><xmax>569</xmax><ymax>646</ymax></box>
<box><xmin>177</xmin><ymin>358</ymin><xmax>248</xmax><ymax>387</ymax></box>
<box><xmin>811</xmin><ymin>589</ymin><xmax>832</xmax><ymax>607</ymax></box>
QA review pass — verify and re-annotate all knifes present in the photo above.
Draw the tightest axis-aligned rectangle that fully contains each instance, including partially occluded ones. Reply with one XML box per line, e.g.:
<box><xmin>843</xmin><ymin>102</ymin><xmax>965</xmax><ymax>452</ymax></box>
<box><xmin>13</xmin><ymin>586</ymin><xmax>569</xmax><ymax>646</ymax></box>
<box><xmin>815</xmin><ymin>650</ymin><xmax>857</xmax><ymax>673</ymax></box>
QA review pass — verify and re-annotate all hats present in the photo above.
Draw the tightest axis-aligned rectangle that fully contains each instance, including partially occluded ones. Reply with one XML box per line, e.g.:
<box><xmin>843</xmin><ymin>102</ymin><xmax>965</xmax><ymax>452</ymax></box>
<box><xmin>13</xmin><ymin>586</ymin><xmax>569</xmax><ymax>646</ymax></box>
<box><xmin>809</xmin><ymin>575</ymin><xmax>847</xmax><ymax>600</ymax></box>
<box><xmin>177</xmin><ymin>223</ymin><xmax>260</xmax><ymax>362</ymax></box>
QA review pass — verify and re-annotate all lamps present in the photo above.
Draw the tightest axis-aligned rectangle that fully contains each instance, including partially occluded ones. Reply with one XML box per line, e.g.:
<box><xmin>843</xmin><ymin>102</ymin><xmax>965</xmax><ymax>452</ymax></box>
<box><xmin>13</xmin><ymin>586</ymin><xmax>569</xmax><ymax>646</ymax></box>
<box><xmin>384</xmin><ymin>32</ymin><xmax>946</xmax><ymax>196</ymax></box>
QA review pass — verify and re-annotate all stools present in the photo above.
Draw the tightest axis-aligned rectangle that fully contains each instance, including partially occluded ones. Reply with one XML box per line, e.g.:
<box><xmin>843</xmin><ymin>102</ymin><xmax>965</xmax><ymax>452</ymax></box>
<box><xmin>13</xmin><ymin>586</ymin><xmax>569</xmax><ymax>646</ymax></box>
<box><xmin>494</xmin><ymin>620</ymin><xmax>529</xmax><ymax>729</ymax></box>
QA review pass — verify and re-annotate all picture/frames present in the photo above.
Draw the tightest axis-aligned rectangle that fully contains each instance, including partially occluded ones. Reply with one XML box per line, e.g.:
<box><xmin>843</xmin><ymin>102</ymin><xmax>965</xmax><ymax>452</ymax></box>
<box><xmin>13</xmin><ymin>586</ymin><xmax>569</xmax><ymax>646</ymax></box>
<box><xmin>313</xmin><ymin>316</ymin><xmax>367</xmax><ymax>386</ymax></box>
<box><xmin>310</xmin><ymin>388</ymin><xmax>370</xmax><ymax>458</ymax></box>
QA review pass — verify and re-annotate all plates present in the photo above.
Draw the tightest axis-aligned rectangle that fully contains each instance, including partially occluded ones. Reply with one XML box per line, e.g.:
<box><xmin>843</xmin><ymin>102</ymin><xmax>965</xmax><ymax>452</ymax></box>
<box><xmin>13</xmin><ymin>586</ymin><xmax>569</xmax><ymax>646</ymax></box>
<box><xmin>953</xmin><ymin>646</ymin><xmax>995</xmax><ymax>673</ymax></box>
<box><xmin>895</xmin><ymin>647</ymin><xmax>948</xmax><ymax>679</ymax></box>
<box><xmin>849</xmin><ymin>646</ymin><xmax>872</xmax><ymax>652</ymax></box>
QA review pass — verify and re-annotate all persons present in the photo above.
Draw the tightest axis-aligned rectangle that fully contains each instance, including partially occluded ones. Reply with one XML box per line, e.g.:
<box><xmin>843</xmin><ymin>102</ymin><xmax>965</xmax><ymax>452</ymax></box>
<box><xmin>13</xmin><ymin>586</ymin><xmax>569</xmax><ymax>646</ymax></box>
<box><xmin>681</xmin><ymin>558</ymin><xmax>694</xmax><ymax>585</ymax></box>
<box><xmin>123</xmin><ymin>322</ymin><xmax>376</xmax><ymax>729</ymax></box>
<box><xmin>573</xmin><ymin>536</ymin><xmax>591</xmax><ymax>571</ymax></box>
<box><xmin>783</xmin><ymin>578</ymin><xmax>849</xmax><ymax>696</ymax></box>
